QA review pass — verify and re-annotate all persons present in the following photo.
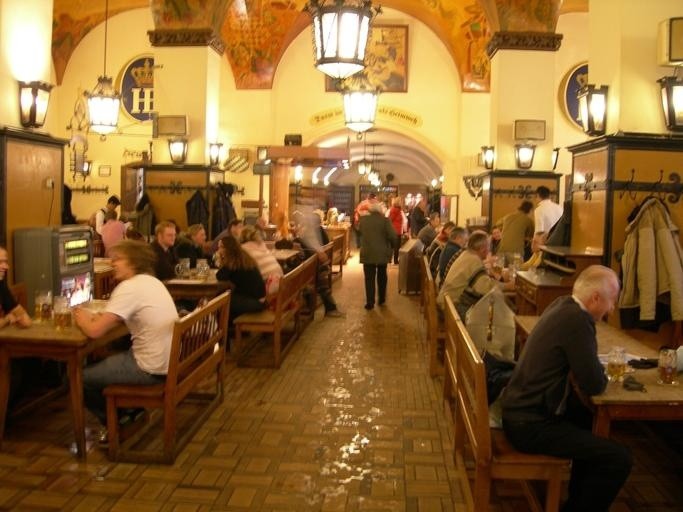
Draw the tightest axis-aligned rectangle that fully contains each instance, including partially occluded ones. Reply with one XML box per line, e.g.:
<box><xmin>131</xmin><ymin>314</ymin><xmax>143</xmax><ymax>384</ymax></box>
<box><xmin>306</xmin><ymin>239</ymin><xmax>344</xmax><ymax>319</ymax></box>
<box><xmin>242</xmin><ymin>224</ymin><xmax>284</xmax><ymax>297</ymax></box>
<box><xmin>177</xmin><ymin>220</ymin><xmax>211</xmax><ymax>250</ymax></box>
<box><xmin>438</xmin><ymin>227</ymin><xmax>468</xmax><ymax>277</ymax></box>
<box><xmin>150</xmin><ymin>221</ymin><xmax>180</xmax><ymax>283</ymax></box>
<box><xmin>101</xmin><ymin>210</ymin><xmax>130</xmax><ymax>256</ymax></box>
<box><xmin>355</xmin><ymin>189</ymin><xmax>387</xmax><ymax>222</ymax></box>
<box><xmin>492</xmin><ymin>227</ymin><xmax>503</xmax><ymax>255</ymax></box>
<box><xmin>436</xmin><ymin>230</ymin><xmax>515</xmax><ymax>321</ymax></box>
<box><xmin>388</xmin><ymin>201</ymin><xmax>403</xmax><ymax>264</ymax></box>
<box><xmin>355</xmin><ymin>202</ymin><xmax>397</xmax><ymax>309</ymax></box>
<box><xmin>494</xmin><ymin>201</ymin><xmax>533</xmax><ymax>253</ymax></box>
<box><xmin>0</xmin><ymin>246</ymin><xmax>31</xmax><ymax>334</ymax></box>
<box><xmin>442</xmin><ymin>243</ymin><xmax>467</xmax><ymax>284</ymax></box>
<box><xmin>216</xmin><ymin>235</ymin><xmax>269</xmax><ymax>354</ymax></box>
<box><xmin>74</xmin><ymin>240</ymin><xmax>178</xmax><ymax>450</ymax></box>
<box><xmin>212</xmin><ymin>219</ymin><xmax>245</xmax><ymax>252</ymax></box>
<box><xmin>500</xmin><ymin>264</ymin><xmax>634</xmax><ymax>507</ymax></box>
<box><xmin>124</xmin><ymin>228</ymin><xmax>146</xmax><ymax>243</ymax></box>
<box><xmin>417</xmin><ymin>212</ymin><xmax>440</xmax><ymax>246</ymax></box>
<box><xmin>410</xmin><ymin>201</ymin><xmax>427</xmax><ymax>236</ymax></box>
<box><xmin>95</xmin><ymin>196</ymin><xmax>120</xmax><ymax>235</ymax></box>
<box><xmin>518</xmin><ymin>232</ymin><xmax>546</xmax><ymax>268</ymax></box>
<box><xmin>438</xmin><ymin>222</ymin><xmax>455</xmax><ymax>243</ymax></box>
<box><xmin>321</xmin><ymin>225</ymin><xmax>331</xmax><ymax>244</ymax></box>
<box><xmin>533</xmin><ymin>186</ymin><xmax>564</xmax><ymax>246</ymax></box>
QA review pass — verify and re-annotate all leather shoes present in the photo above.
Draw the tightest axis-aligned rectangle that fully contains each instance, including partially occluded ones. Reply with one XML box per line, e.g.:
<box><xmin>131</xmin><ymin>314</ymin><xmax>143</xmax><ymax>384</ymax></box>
<box><xmin>379</xmin><ymin>298</ymin><xmax>386</xmax><ymax>305</ymax></box>
<box><xmin>365</xmin><ymin>303</ymin><xmax>375</xmax><ymax>310</ymax></box>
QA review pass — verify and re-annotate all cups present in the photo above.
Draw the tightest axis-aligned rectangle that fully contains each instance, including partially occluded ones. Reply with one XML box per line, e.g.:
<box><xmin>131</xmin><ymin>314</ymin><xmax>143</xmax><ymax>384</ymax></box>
<box><xmin>608</xmin><ymin>345</ymin><xmax>627</xmax><ymax>384</ymax></box>
<box><xmin>174</xmin><ymin>258</ymin><xmax>190</xmax><ymax>278</ymax></box>
<box><xmin>34</xmin><ymin>288</ymin><xmax>52</xmax><ymax>322</ymax></box>
<box><xmin>657</xmin><ymin>349</ymin><xmax>677</xmax><ymax>384</ymax></box>
<box><xmin>197</xmin><ymin>263</ymin><xmax>209</xmax><ymax>279</ymax></box>
<box><xmin>54</xmin><ymin>295</ymin><xmax>72</xmax><ymax>329</ymax></box>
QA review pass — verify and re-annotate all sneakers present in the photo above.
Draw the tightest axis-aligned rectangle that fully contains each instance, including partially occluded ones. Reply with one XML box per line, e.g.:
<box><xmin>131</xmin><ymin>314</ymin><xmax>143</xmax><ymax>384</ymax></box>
<box><xmin>132</xmin><ymin>410</ymin><xmax>145</xmax><ymax>422</ymax></box>
<box><xmin>97</xmin><ymin>414</ymin><xmax>132</xmax><ymax>446</ymax></box>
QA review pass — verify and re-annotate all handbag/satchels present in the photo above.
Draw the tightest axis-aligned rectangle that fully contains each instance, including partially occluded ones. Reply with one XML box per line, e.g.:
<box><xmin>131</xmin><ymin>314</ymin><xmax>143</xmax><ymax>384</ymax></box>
<box><xmin>481</xmin><ymin>349</ymin><xmax>516</xmax><ymax>406</ymax></box>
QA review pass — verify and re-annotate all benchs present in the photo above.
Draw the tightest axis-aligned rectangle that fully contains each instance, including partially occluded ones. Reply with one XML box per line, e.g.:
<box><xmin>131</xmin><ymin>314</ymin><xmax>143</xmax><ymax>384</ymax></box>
<box><xmin>442</xmin><ymin>292</ymin><xmax>572</xmax><ymax>512</ymax></box>
<box><xmin>104</xmin><ymin>289</ymin><xmax>235</xmax><ymax>465</ymax></box>
<box><xmin>95</xmin><ymin>268</ymin><xmax>119</xmax><ymax>299</ymax></box>
<box><xmin>422</xmin><ymin>254</ymin><xmax>446</xmax><ymax>376</ymax></box>
<box><xmin>233</xmin><ymin>252</ymin><xmax>320</xmax><ymax>371</ymax></box>
<box><xmin>312</xmin><ymin>240</ymin><xmax>335</xmax><ymax>310</ymax></box>
<box><xmin>332</xmin><ymin>232</ymin><xmax>346</xmax><ymax>286</ymax></box>
<box><xmin>11</xmin><ymin>280</ymin><xmax>30</xmax><ymax>313</ymax></box>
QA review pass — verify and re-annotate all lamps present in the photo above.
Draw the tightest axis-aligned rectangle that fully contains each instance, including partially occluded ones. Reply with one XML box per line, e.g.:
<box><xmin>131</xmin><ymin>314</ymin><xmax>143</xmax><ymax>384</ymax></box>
<box><xmin>82</xmin><ymin>0</ymin><xmax>125</xmax><ymax>137</ymax></box>
<box><xmin>480</xmin><ymin>145</ymin><xmax>494</xmax><ymax>170</ymax></box>
<box><xmin>302</xmin><ymin>0</ymin><xmax>382</xmax><ymax>83</ymax></box>
<box><xmin>166</xmin><ymin>137</ymin><xmax>186</xmax><ymax>164</ymax></box>
<box><xmin>356</xmin><ymin>129</ymin><xmax>374</xmax><ymax>175</ymax></box>
<box><xmin>18</xmin><ymin>79</ymin><xmax>54</xmax><ymax>129</ymax></box>
<box><xmin>658</xmin><ymin>75</ymin><xmax>683</xmax><ymax>131</ymax></box>
<box><xmin>342</xmin><ymin>88</ymin><xmax>379</xmax><ymax>142</ymax></box>
<box><xmin>578</xmin><ymin>81</ymin><xmax>610</xmax><ymax>136</ymax></box>
<box><xmin>516</xmin><ymin>142</ymin><xmax>536</xmax><ymax>169</ymax></box>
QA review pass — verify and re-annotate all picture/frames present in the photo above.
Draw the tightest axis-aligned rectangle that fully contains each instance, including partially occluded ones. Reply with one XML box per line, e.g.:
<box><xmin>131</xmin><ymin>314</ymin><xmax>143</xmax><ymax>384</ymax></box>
<box><xmin>324</xmin><ymin>24</ymin><xmax>410</xmax><ymax>93</ymax></box>
